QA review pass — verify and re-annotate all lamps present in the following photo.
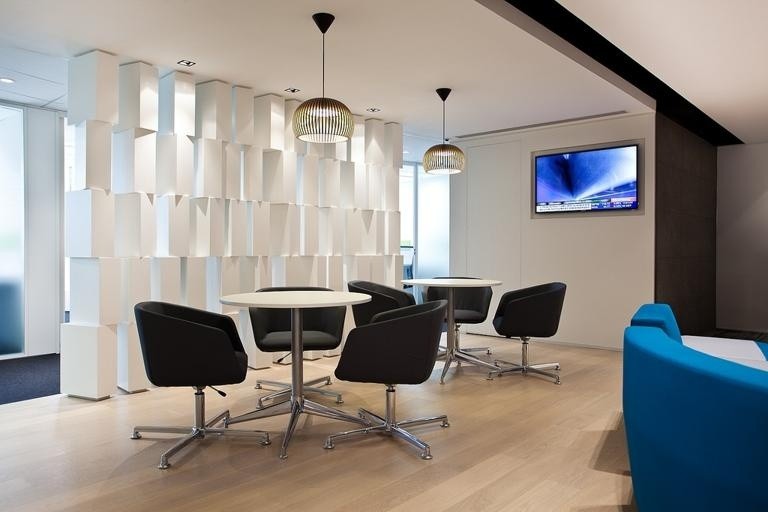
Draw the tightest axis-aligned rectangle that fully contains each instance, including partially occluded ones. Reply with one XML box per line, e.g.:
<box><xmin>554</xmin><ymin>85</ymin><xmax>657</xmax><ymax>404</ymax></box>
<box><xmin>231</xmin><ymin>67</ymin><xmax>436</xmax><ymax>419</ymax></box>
<box><xmin>422</xmin><ymin>88</ymin><xmax>465</xmax><ymax>176</ymax></box>
<box><xmin>291</xmin><ymin>13</ymin><xmax>355</xmax><ymax>144</ymax></box>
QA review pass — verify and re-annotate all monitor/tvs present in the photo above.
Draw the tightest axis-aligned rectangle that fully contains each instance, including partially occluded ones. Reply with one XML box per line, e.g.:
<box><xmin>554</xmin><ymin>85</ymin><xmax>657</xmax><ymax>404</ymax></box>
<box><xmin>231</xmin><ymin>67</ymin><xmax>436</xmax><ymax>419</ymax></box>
<box><xmin>530</xmin><ymin>137</ymin><xmax>645</xmax><ymax>220</ymax></box>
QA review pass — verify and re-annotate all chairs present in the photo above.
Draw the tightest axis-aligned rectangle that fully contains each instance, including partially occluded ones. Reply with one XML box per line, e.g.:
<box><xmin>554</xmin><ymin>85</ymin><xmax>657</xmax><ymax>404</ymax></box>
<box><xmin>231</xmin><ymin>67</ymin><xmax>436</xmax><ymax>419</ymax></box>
<box><xmin>421</xmin><ymin>277</ymin><xmax>492</xmax><ymax>363</ymax></box>
<box><xmin>622</xmin><ymin>324</ymin><xmax>768</xmax><ymax>512</ymax></box>
<box><xmin>323</xmin><ymin>300</ymin><xmax>449</xmax><ymax>460</ymax></box>
<box><xmin>248</xmin><ymin>287</ymin><xmax>346</xmax><ymax>409</ymax></box>
<box><xmin>632</xmin><ymin>303</ymin><xmax>768</xmax><ymax>371</ymax></box>
<box><xmin>347</xmin><ymin>279</ymin><xmax>415</xmax><ymax>328</ymax></box>
<box><xmin>487</xmin><ymin>282</ymin><xmax>566</xmax><ymax>385</ymax></box>
<box><xmin>129</xmin><ymin>301</ymin><xmax>272</xmax><ymax>469</ymax></box>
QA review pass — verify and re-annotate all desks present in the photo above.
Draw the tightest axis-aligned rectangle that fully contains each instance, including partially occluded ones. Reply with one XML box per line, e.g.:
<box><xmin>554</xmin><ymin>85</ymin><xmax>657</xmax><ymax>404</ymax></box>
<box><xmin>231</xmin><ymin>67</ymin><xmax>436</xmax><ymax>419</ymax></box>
<box><xmin>401</xmin><ymin>278</ymin><xmax>502</xmax><ymax>384</ymax></box>
<box><xmin>219</xmin><ymin>291</ymin><xmax>372</xmax><ymax>460</ymax></box>
